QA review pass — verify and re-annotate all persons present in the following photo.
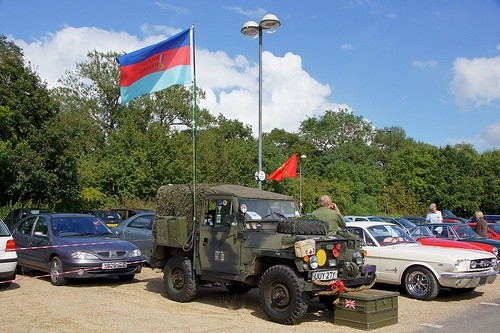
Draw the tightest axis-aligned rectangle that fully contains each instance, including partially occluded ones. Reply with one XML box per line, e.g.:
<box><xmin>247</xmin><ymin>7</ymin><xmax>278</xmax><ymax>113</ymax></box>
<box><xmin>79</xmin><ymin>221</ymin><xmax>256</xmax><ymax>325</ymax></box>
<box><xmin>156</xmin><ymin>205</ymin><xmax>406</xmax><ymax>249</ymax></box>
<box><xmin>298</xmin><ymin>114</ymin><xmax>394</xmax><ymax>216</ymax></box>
<box><xmin>311</xmin><ymin>195</ymin><xmax>346</xmax><ymax>236</ymax></box>
<box><xmin>475</xmin><ymin>211</ymin><xmax>489</xmax><ymax>238</ymax></box>
<box><xmin>425</xmin><ymin>204</ymin><xmax>443</xmax><ymax>234</ymax></box>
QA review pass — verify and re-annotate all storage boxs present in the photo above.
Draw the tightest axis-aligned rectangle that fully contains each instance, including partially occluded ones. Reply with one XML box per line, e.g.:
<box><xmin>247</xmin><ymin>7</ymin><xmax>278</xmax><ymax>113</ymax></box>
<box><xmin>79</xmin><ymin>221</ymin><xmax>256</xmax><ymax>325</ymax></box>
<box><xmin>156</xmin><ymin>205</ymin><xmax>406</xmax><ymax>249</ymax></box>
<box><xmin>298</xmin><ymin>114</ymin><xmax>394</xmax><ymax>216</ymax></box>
<box><xmin>334</xmin><ymin>290</ymin><xmax>400</xmax><ymax>331</ymax></box>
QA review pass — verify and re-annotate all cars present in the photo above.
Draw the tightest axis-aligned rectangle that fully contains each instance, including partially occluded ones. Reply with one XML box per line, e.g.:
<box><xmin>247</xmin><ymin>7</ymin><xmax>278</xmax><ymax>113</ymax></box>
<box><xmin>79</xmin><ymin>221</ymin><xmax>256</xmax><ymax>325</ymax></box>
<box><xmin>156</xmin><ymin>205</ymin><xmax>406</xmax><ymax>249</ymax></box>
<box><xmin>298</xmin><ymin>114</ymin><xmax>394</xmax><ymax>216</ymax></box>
<box><xmin>0</xmin><ymin>208</ymin><xmax>154</xmax><ymax>289</ymax></box>
<box><xmin>10</xmin><ymin>213</ymin><xmax>144</xmax><ymax>286</ymax></box>
<box><xmin>344</xmin><ymin>221</ymin><xmax>499</xmax><ymax>301</ymax></box>
<box><xmin>344</xmin><ymin>213</ymin><xmax>500</xmax><ymax>255</ymax></box>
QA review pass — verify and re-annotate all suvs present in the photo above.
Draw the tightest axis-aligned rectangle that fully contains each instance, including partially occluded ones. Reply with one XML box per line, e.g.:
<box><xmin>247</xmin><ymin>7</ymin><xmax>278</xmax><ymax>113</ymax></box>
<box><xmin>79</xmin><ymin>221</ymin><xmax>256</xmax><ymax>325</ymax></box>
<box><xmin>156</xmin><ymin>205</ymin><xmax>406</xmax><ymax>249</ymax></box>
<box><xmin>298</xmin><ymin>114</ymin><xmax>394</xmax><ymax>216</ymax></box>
<box><xmin>150</xmin><ymin>183</ymin><xmax>377</xmax><ymax>326</ymax></box>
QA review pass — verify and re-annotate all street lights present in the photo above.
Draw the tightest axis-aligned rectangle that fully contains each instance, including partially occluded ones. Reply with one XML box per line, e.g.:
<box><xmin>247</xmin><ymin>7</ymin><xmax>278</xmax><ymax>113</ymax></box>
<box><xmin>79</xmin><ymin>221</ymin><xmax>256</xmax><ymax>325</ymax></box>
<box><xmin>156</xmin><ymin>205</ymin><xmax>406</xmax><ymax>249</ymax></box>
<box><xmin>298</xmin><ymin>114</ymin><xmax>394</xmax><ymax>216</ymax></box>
<box><xmin>241</xmin><ymin>14</ymin><xmax>281</xmax><ymax>191</ymax></box>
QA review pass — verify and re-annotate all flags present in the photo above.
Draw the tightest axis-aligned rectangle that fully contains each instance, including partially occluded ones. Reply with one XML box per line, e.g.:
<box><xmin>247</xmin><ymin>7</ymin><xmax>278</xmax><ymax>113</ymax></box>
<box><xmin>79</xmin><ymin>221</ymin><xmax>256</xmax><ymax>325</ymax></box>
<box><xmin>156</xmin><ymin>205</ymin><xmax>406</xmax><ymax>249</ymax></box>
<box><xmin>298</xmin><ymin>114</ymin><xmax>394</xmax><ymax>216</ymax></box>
<box><xmin>268</xmin><ymin>153</ymin><xmax>297</xmax><ymax>181</ymax></box>
<box><xmin>116</xmin><ymin>28</ymin><xmax>193</xmax><ymax>105</ymax></box>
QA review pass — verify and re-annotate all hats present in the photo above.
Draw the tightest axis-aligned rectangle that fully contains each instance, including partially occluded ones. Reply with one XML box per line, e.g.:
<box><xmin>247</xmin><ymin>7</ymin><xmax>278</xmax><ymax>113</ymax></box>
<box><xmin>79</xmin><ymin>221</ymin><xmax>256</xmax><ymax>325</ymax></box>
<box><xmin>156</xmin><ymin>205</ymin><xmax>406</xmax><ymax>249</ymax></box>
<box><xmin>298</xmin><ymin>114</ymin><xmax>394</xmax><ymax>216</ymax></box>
<box><xmin>429</xmin><ymin>203</ymin><xmax>436</xmax><ymax>210</ymax></box>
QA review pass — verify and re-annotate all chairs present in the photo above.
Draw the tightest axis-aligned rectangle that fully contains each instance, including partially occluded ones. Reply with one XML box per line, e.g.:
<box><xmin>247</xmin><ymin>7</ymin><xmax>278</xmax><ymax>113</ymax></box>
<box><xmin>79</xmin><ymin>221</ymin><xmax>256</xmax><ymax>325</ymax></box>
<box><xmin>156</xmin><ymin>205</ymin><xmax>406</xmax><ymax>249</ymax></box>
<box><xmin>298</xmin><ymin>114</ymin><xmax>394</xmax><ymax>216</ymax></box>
<box><xmin>57</xmin><ymin>223</ymin><xmax>66</xmax><ymax>232</ymax></box>
<box><xmin>37</xmin><ymin>225</ymin><xmax>48</xmax><ymax>233</ymax></box>
<box><xmin>441</xmin><ymin>230</ymin><xmax>448</xmax><ymax>236</ymax></box>
<box><xmin>80</xmin><ymin>220</ymin><xmax>95</xmax><ymax>232</ymax></box>
